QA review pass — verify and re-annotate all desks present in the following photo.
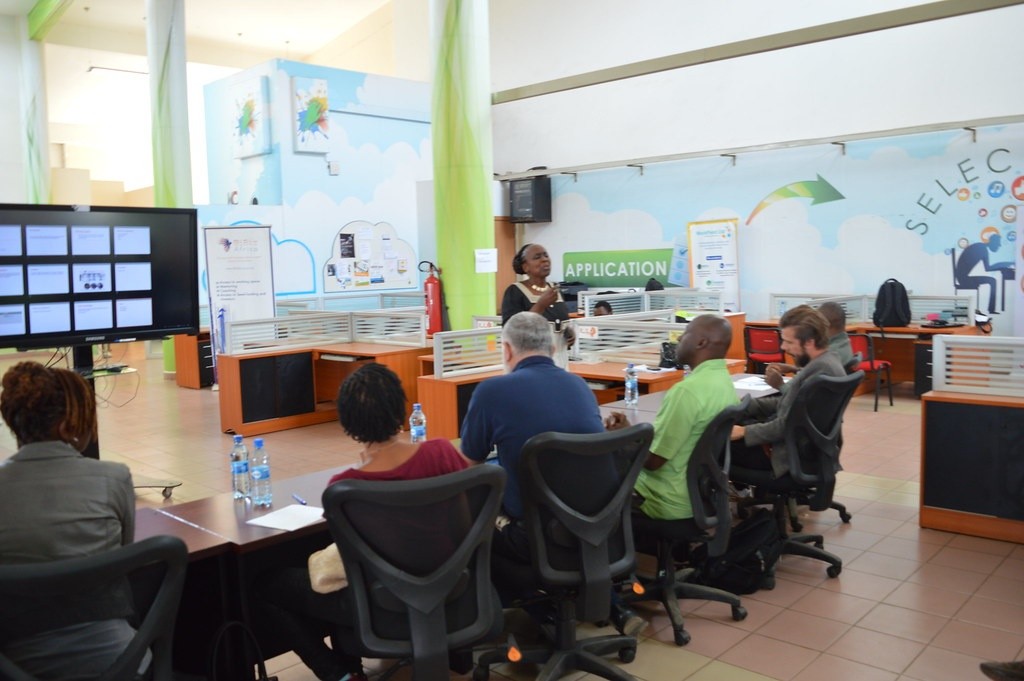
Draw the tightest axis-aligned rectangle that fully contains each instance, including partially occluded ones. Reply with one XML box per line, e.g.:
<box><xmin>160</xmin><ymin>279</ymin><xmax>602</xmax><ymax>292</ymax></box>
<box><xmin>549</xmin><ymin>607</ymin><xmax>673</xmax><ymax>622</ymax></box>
<box><xmin>125</xmin><ymin>318</ymin><xmax>1024</xmax><ymax>681</ymax></box>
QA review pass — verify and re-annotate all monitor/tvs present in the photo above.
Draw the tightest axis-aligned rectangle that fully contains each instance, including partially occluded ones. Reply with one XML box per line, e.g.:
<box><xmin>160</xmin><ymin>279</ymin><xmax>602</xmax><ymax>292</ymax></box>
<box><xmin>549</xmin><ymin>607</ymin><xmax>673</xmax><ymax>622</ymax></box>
<box><xmin>0</xmin><ymin>203</ymin><xmax>201</xmax><ymax>350</ymax></box>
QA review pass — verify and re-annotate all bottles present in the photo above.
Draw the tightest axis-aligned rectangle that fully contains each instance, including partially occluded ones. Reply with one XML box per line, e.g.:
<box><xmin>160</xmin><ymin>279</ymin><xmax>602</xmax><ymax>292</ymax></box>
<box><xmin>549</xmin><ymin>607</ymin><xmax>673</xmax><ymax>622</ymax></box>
<box><xmin>625</xmin><ymin>363</ymin><xmax>638</xmax><ymax>403</ymax></box>
<box><xmin>229</xmin><ymin>434</ymin><xmax>251</xmax><ymax>500</ymax></box>
<box><xmin>683</xmin><ymin>365</ymin><xmax>691</xmax><ymax>378</ymax></box>
<box><xmin>408</xmin><ymin>404</ymin><xmax>426</xmax><ymax>443</ymax></box>
<box><xmin>249</xmin><ymin>438</ymin><xmax>272</xmax><ymax>504</ymax></box>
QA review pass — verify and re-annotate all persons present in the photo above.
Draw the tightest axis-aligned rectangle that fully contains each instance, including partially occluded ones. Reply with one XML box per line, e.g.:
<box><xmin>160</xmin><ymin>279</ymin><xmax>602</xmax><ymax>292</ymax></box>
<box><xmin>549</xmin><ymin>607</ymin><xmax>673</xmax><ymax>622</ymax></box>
<box><xmin>765</xmin><ymin>301</ymin><xmax>854</xmax><ymax>392</ymax></box>
<box><xmin>459</xmin><ymin>312</ymin><xmax>649</xmax><ymax>633</ymax></box>
<box><xmin>502</xmin><ymin>244</ymin><xmax>575</xmax><ymax>350</ymax></box>
<box><xmin>594</xmin><ymin>301</ymin><xmax>612</xmax><ymax>316</ymax></box>
<box><xmin>608</xmin><ymin>314</ymin><xmax>738</xmax><ymax>585</ymax></box>
<box><xmin>257</xmin><ymin>363</ymin><xmax>469</xmax><ymax>681</ymax></box>
<box><xmin>0</xmin><ymin>361</ymin><xmax>152</xmax><ymax>681</ymax></box>
<box><xmin>732</xmin><ymin>305</ymin><xmax>847</xmax><ymax>542</ymax></box>
<box><xmin>646</xmin><ymin>278</ymin><xmax>663</xmax><ymax>291</ymax></box>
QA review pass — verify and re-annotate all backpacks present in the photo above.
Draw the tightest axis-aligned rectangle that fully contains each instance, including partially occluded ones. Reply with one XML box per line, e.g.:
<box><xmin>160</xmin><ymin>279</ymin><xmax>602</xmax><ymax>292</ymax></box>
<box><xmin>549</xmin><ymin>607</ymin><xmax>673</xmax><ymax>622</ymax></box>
<box><xmin>873</xmin><ymin>279</ymin><xmax>911</xmax><ymax>327</ymax></box>
<box><xmin>689</xmin><ymin>506</ymin><xmax>783</xmax><ymax>596</ymax></box>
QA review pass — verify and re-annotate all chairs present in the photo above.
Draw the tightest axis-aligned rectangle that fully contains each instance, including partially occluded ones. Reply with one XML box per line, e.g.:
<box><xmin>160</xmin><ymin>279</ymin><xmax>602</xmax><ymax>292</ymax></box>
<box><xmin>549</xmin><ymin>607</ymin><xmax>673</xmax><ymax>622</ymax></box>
<box><xmin>845</xmin><ymin>333</ymin><xmax>895</xmax><ymax>412</ymax></box>
<box><xmin>743</xmin><ymin>325</ymin><xmax>785</xmax><ymax>376</ymax></box>
<box><xmin>778</xmin><ymin>350</ymin><xmax>863</xmax><ymax>531</ymax></box>
<box><xmin>473</xmin><ymin>421</ymin><xmax>655</xmax><ymax>680</ymax></box>
<box><xmin>320</xmin><ymin>464</ymin><xmax>506</xmax><ymax>680</ymax></box>
<box><xmin>619</xmin><ymin>394</ymin><xmax>749</xmax><ymax>647</ymax></box>
<box><xmin>745</xmin><ymin>370</ymin><xmax>865</xmax><ymax>590</ymax></box>
<box><xmin>0</xmin><ymin>536</ymin><xmax>190</xmax><ymax>681</ymax></box>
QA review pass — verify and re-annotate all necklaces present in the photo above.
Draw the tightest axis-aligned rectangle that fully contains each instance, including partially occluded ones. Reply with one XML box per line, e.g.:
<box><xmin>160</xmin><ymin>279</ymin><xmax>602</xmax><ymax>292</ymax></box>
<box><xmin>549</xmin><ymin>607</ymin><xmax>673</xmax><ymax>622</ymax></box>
<box><xmin>528</xmin><ymin>279</ymin><xmax>548</xmax><ymax>292</ymax></box>
<box><xmin>367</xmin><ymin>440</ymin><xmax>397</xmax><ymax>454</ymax></box>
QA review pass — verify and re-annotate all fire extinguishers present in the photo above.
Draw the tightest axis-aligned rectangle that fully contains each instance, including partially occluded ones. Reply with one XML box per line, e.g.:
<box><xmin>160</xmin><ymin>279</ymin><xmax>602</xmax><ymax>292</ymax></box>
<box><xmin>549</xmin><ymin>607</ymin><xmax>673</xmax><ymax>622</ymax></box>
<box><xmin>418</xmin><ymin>260</ymin><xmax>442</xmax><ymax>334</ymax></box>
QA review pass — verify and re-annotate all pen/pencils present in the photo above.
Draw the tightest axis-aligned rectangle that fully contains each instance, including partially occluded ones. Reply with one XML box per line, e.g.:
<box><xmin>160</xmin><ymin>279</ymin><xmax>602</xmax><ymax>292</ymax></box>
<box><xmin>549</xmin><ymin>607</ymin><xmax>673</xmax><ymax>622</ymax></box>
<box><xmin>546</xmin><ymin>282</ymin><xmax>551</xmax><ymax>288</ymax></box>
<box><xmin>293</xmin><ymin>495</ymin><xmax>306</xmax><ymax>505</ymax></box>
<box><xmin>763</xmin><ymin>363</ymin><xmax>770</xmax><ymax>364</ymax></box>
